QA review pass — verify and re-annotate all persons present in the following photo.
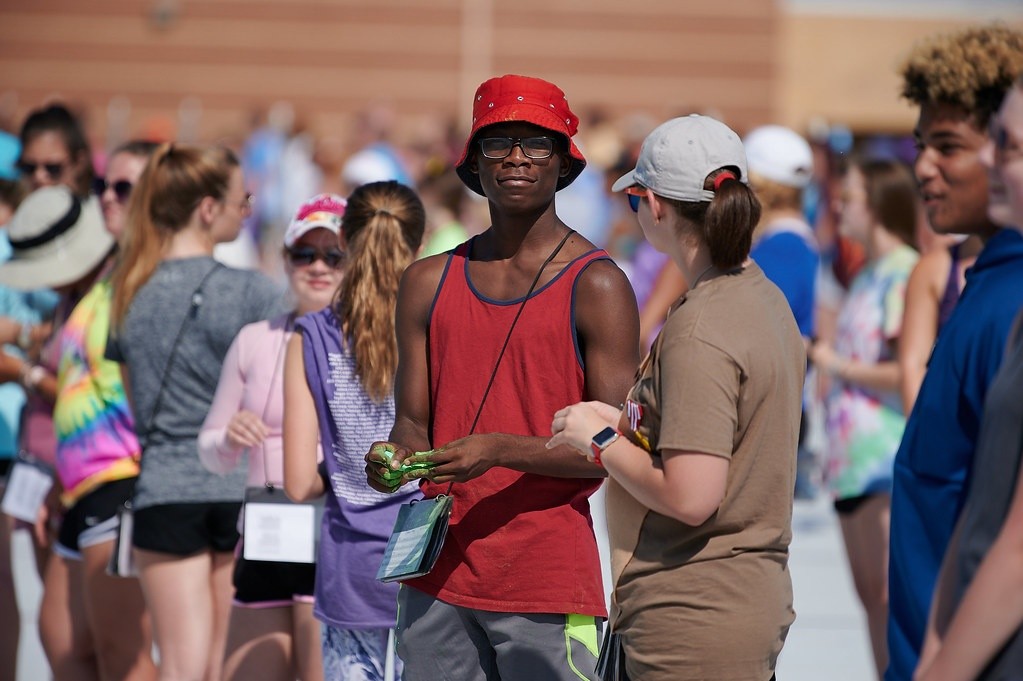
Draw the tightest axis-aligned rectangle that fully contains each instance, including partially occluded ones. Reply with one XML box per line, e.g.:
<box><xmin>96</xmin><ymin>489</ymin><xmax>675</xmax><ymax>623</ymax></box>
<box><xmin>282</xmin><ymin>180</ymin><xmax>428</xmax><ymax>680</ymax></box>
<box><xmin>1</xmin><ymin>102</ymin><xmax>117</xmax><ymax>681</ymax></box>
<box><xmin>885</xmin><ymin>18</ymin><xmax>1023</xmax><ymax>680</ymax></box>
<box><xmin>103</xmin><ymin>139</ymin><xmax>294</xmax><ymax>681</ymax></box>
<box><xmin>911</xmin><ymin>73</ymin><xmax>1023</xmax><ymax>681</ymax></box>
<box><xmin>548</xmin><ymin>112</ymin><xmax>808</xmax><ymax>681</ymax></box>
<box><xmin>197</xmin><ymin>190</ymin><xmax>348</xmax><ymax>680</ymax></box>
<box><xmin>39</xmin><ymin>138</ymin><xmax>160</xmax><ymax>681</ymax></box>
<box><xmin>739</xmin><ymin>124</ymin><xmax>822</xmax><ymax>453</ymax></box>
<box><xmin>809</xmin><ymin>152</ymin><xmax>922</xmax><ymax>681</ymax></box>
<box><xmin>365</xmin><ymin>74</ymin><xmax>641</xmax><ymax>679</ymax></box>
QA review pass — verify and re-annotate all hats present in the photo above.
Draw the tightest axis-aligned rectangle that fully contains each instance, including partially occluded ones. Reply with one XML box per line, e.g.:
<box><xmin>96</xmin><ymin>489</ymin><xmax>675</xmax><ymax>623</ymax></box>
<box><xmin>611</xmin><ymin>113</ymin><xmax>748</xmax><ymax>202</ymax></box>
<box><xmin>284</xmin><ymin>195</ymin><xmax>348</xmax><ymax>244</ymax></box>
<box><xmin>741</xmin><ymin>125</ymin><xmax>814</xmax><ymax>190</ymax></box>
<box><xmin>0</xmin><ymin>183</ymin><xmax>117</xmax><ymax>291</ymax></box>
<box><xmin>454</xmin><ymin>75</ymin><xmax>587</xmax><ymax>197</ymax></box>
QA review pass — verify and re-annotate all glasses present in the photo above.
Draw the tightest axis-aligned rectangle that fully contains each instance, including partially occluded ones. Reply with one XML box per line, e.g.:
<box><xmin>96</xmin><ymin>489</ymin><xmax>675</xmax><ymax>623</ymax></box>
<box><xmin>286</xmin><ymin>243</ymin><xmax>346</xmax><ymax>269</ymax></box>
<box><xmin>624</xmin><ymin>187</ymin><xmax>648</xmax><ymax>213</ymax></box>
<box><xmin>92</xmin><ymin>178</ymin><xmax>131</xmax><ymax>202</ymax></box>
<box><xmin>15</xmin><ymin>161</ymin><xmax>69</xmax><ymax>179</ymax></box>
<box><xmin>478</xmin><ymin>136</ymin><xmax>558</xmax><ymax>159</ymax></box>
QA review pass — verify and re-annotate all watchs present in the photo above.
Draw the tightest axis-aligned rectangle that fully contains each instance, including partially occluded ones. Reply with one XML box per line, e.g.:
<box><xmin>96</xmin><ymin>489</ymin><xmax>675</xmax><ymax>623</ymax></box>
<box><xmin>590</xmin><ymin>426</ymin><xmax>622</xmax><ymax>467</ymax></box>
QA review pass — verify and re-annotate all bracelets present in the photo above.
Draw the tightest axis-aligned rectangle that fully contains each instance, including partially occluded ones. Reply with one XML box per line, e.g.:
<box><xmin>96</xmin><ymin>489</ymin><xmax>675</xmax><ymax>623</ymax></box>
<box><xmin>18</xmin><ymin>324</ymin><xmax>32</xmax><ymax>350</ymax></box>
<box><xmin>22</xmin><ymin>365</ymin><xmax>48</xmax><ymax>389</ymax></box>
